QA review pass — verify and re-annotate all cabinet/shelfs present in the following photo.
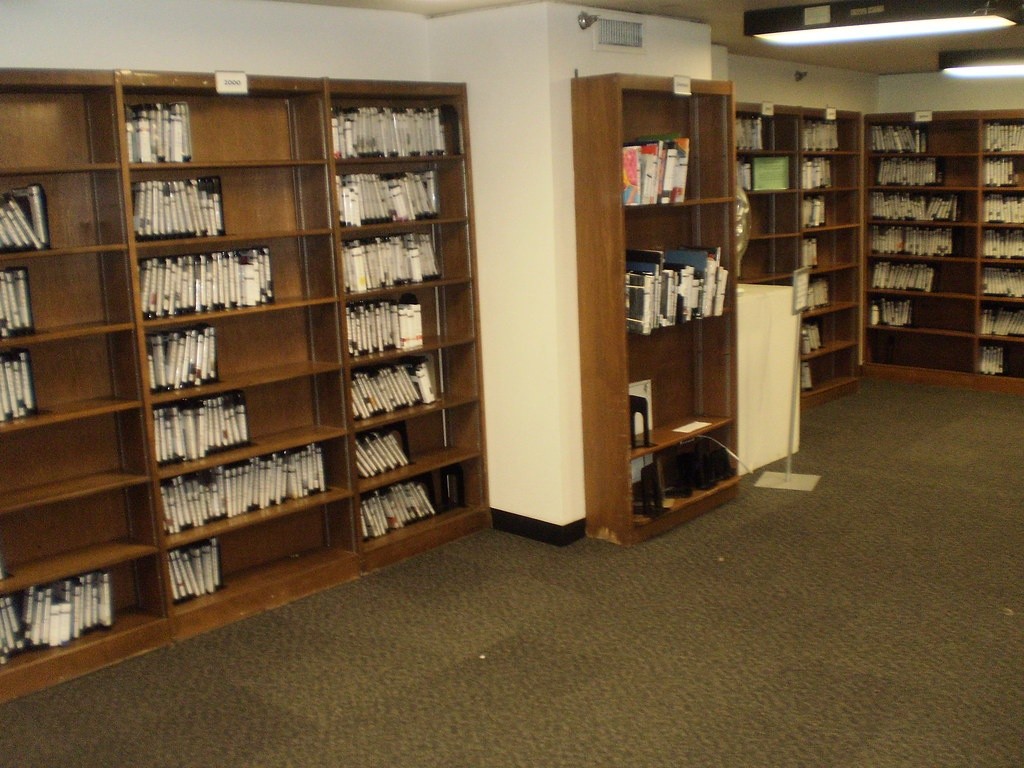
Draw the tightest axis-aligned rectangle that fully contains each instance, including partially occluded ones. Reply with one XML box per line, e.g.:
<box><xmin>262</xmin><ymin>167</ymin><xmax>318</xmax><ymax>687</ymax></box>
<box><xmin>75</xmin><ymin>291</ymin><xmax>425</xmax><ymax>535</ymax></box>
<box><xmin>733</xmin><ymin>100</ymin><xmax>864</xmax><ymax>398</ymax></box>
<box><xmin>863</xmin><ymin>110</ymin><xmax>1024</xmax><ymax>393</ymax></box>
<box><xmin>734</xmin><ymin>284</ymin><xmax>804</xmax><ymax>472</ymax></box>
<box><xmin>569</xmin><ymin>71</ymin><xmax>742</xmax><ymax>549</ymax></box>
<box><xmin>0</xmin><ymin>66</ymin><xmax>492</xmax><ymax>706</ymax></box>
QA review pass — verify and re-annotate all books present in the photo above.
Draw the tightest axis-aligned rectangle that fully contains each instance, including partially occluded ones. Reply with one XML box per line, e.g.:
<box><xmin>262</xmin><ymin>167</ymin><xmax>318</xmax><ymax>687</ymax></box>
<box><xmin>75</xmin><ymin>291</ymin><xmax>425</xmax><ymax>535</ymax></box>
<box><xmin>618</xmin><ymin>132</ymin><xmax>730</xmax><ymax>335</ymax></box>
<box><xmin>0</xmin><ymin>184</ymin><xmax>52</xmax><ymax>253</ymax></box>
<box><xmin>0</xmin><ymin>350</ymin><xmax>38</xmax><ymax>422</ymax></box>
<box><xmin>1</xmin><ymin>571</ymin><xmax>116</xmax><ymax>668</ymax></box>
<box><xmin>2</xmin><ymin>268</ymin><xmax>38</xmax><ymax>340</ymax></box>
<box><xmin>123</xmin><ymin>99</ymin><xmax>465</xmax><ymax>603</ymax></box>
<box><xmin>800</xmin><ymin>120</ymin><xmax>841</xmax><ymax>390</ymax></box>
<box><xmin>732</xmin><ymin>113</ymin><xmax>770</xmax><ymax>153</ymax></box>
<box><xmin>865</xmin><ymin>123</ymin><xmax>1024</xmax><ymax>378</ymax></box>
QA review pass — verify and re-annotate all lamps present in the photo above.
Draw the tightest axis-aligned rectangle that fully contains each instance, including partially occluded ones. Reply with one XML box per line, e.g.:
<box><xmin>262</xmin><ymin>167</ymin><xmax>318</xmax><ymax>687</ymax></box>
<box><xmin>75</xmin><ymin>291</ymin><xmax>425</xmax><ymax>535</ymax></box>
<box><xmin>742</xmin><ymin>0</ymin><xmax>1024</xmax><ymax>47</ymax></box>
<box><xmin>938</xmin><ymin>48</ymin><xmax>1024</xmax><ymax>79</ymax></box>
